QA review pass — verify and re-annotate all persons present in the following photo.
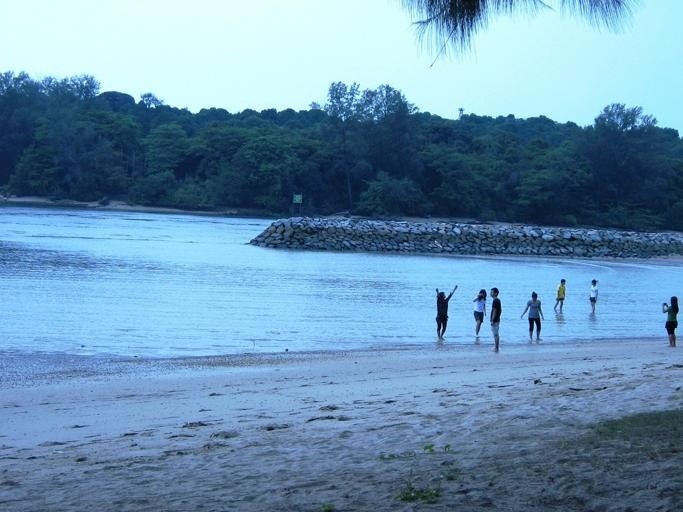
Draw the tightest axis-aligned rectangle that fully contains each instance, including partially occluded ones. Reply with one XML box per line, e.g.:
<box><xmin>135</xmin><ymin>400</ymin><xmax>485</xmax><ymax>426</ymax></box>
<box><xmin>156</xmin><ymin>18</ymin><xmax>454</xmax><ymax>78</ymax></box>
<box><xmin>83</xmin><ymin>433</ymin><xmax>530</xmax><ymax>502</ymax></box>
<box><xmin>471</xmin><ymin>288</ymin><xmax>487</xmax><ymax>339</ymax></box>
<box><xmin>587</xmin><ymin>279</ymin><xmax>599</xmax><ymax>313</ymax></box>
<box><xmin>434</xmin><ymin>284</ymin><xmax>458</xmax><ymax>341</ymax></box>
<box><xmin>520</xmin><ymin>290</ymin><xmax>545</xmax><ymax>342</ymax></box>
<box><xmin>554</xmin><ymin>311</ymin><xmax>565</xmax><ymax>332</ymax></box>
<box><xmin>660</xmin><ymin>296</ymin><xmax>679</xmax><ymax>348</ymax></box>
<box><xmin>587</xmin><ymin>314</ymin><xmax>599</xmax><ymax>324</ymax></box>
<box><xmin>488</xmin><ymin>287</ymin><xmax>502</xmax><ymax>353</ymax></box>
<box><xmin>552</xmin><ymin>279</ymin><xmax>566</xmax><ymax>311</ymax></box>
